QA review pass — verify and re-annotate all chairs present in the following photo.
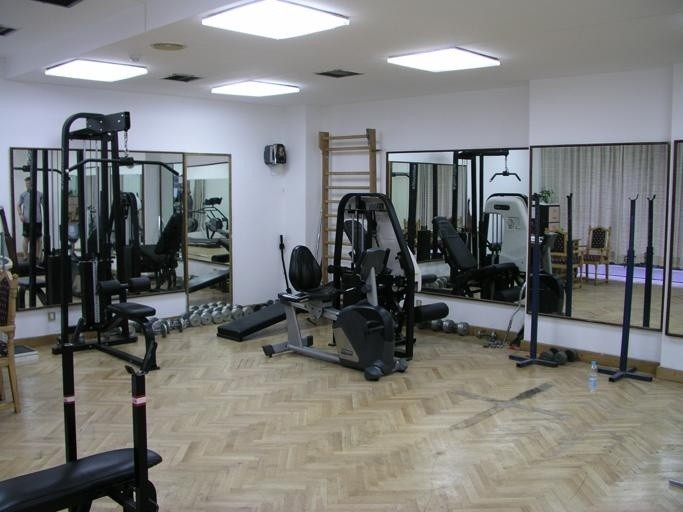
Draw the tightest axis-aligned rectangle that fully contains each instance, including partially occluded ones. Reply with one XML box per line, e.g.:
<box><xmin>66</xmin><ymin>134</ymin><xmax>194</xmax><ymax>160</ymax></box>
<box><xmin>0</xmin><ymin>264</ymin><xmax>22</xmax><ymax>415</ymax></box>
<box><xmin>288</xmin><ymin>244</ymin><xmax>337</xmax><ymax>299</ymax></box>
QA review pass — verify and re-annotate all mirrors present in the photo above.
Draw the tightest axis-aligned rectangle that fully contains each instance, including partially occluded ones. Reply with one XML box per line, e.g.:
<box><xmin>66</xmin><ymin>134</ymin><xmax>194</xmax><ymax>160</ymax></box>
<box><xmin>9</xmin><ymin>146</ymin><xmax>234</xmax><ymax>316</ymax></box>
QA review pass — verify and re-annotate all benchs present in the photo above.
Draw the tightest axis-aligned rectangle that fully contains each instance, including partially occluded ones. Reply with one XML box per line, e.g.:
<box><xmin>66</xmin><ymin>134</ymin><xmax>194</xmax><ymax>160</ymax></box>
<box><xmin>0</xmin><ymin>448</ymin><xmax>162</xmax><ymax>512</ymax></box>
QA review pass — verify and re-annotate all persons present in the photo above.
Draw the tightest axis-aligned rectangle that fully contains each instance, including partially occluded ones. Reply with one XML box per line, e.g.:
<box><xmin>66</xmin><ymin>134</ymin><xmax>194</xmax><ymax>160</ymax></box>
<box><xmin>17</xmin><ymin>176</ymin><xmax>44</xmax><ymax>260</ymax></box>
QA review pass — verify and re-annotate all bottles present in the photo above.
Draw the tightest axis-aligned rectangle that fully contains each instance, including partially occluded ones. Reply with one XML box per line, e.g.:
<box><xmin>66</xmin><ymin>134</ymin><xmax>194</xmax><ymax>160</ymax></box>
<box><xmin>588</xmin><ymin>361</ymin><xmax>599</xmax><ymax>396</ymax></box>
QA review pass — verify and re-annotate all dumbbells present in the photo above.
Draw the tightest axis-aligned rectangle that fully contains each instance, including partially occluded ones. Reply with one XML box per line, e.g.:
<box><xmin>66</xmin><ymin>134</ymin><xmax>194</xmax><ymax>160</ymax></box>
<box><xmin>117</xmin><ymin>301</ymin><xmax>255</xmax><ymax>337</ymax></box>
<box><xmin>539</xmin><ymin>347</ymin><xmax>577</xmax><ymax>365</ymax></box>
<box><xmin>417</xmin><ymin>319</ymin><xmax>497</xmax><ymax>338</ymax></box>
